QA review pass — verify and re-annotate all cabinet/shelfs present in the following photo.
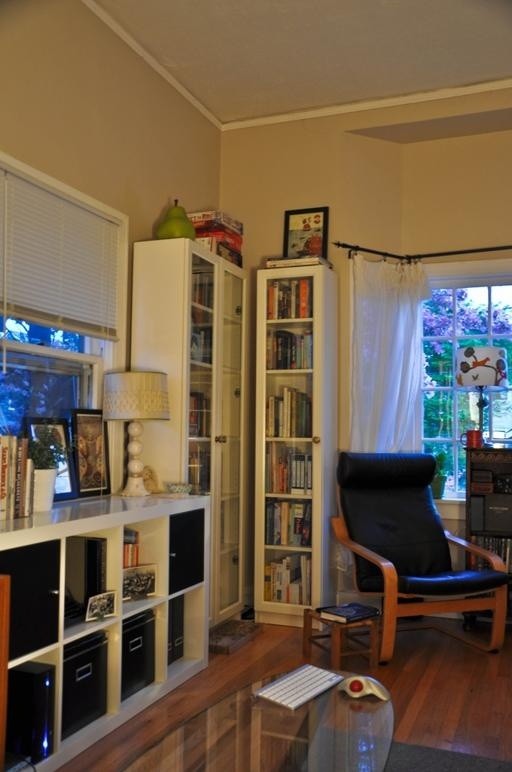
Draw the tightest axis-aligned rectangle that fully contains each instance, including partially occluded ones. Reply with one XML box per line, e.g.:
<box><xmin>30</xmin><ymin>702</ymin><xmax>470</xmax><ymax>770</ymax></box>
<box><xmin>0</xmin><ymin>495</ymin><xmax>211</xmax><ymax>772</ymax></box>
<box><xmin>462</xmin><ymin>447</ymin><xmax>512</xmax><ymax>622</ymax></box>
<box><xmin>130</xmin><ymin>237</ymin><xmax>252</xmax><ymax>628</ymax></box>
<box><xmin>254</xmin><ymin>264</ymin><xmax>339</xmax><ymax>633</ymax></box>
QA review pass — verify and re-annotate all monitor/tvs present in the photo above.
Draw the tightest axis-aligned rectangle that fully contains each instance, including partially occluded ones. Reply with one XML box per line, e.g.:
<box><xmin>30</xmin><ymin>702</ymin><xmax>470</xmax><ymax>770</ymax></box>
<box><xmin>64</xmin><ymin>536</ymin><xmax>89</xmax><ymax>630</ymax></box>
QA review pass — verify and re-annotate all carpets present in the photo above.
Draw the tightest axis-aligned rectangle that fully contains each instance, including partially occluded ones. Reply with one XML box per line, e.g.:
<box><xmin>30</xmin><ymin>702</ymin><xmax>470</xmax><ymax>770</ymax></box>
<box><xmin>301</xmin><ymin>723</ymin><xmax>512</xmax><ymax>772</ymax></box>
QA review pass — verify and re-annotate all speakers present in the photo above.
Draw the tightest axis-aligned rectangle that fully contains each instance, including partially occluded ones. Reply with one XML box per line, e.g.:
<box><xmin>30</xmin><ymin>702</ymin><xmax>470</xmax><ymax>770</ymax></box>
<box><xmin>122</xmin><ymin>609</ymin><xmax>155</xmax><ymax>704</ymax></box>
<box><xmin>62</xmin><ymin>633</ymin><xmax>109</xmax><ymax>743</ymax></box>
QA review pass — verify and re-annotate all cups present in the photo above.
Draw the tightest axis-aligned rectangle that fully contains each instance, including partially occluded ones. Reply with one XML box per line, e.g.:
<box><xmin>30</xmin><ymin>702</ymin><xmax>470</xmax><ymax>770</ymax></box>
<box><xmin>460</xmin><ymin>430</ymin><xmax>482</xmax><ymax>449</ymax></box>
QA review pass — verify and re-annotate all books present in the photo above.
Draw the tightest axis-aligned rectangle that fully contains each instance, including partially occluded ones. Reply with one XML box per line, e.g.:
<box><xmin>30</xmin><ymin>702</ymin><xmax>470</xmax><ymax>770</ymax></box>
<box><xmin>191</xmin><ymin>261</ymin><xmax>211</xmax><ymax>493</ymax></box>
<box><xmin>0</xmin><ymin>435</ymin><xmax>33</xmax><ymax>520</ymax></box>
<box><xmin>320</xmin><ymin>599</ymin><xmax>382</xmax><ymax>625</ymax></box>
<box><xmin>465</xmin><ymin>533</ymin><xmax>511</xmax><ymax>603</ymax></box>
<box><xmin>266</xmin><ymin>275</ymin><xmax>315</xmax><ymax>604</ymax></box>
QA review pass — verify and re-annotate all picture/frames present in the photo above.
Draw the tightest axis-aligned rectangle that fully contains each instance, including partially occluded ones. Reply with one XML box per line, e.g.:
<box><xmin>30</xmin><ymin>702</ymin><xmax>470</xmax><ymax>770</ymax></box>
<box><xmin>72</xmin><ymin>409</ymin><xmax>111</xmax><ymax>498</ymax></box>
<box><xmin>24</xmin><ymin>417</ymin><xmax>78</xmax><ymax>501</ymax></box>
<box><xmin>284</xmin><ymin>207</ymin><xmax>328</xmax><ymax>259</ymax></box>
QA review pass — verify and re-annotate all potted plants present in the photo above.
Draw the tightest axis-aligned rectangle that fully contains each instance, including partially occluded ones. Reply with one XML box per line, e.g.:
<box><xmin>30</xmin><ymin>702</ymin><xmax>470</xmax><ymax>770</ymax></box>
<box><xmin>21</xmin><ymin>416</ymin><xmax>76</xmax><ymax>511</ymax></box>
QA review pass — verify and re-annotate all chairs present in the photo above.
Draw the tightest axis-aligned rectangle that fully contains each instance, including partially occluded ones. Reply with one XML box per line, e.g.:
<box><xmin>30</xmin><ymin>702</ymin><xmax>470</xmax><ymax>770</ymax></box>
<box><xmin>330</xmin><ymin>452</ymin><xmax>512</xmax><ymax>664</ymax></box>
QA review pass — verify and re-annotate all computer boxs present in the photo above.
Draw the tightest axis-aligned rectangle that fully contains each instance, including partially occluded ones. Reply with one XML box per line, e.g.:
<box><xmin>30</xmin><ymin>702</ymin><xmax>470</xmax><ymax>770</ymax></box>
<box><xmin>9</xmin><ymin>662</ymin><xmax>56</xmax><ymax>767</ymax></box>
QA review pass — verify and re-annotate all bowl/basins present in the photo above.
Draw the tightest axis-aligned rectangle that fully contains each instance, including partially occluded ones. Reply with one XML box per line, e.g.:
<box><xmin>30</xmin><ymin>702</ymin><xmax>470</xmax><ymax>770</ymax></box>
<box><xmin>168</xmin><ymin>483</ymin><xmax>193</xmax><ymax>494</ymax></box>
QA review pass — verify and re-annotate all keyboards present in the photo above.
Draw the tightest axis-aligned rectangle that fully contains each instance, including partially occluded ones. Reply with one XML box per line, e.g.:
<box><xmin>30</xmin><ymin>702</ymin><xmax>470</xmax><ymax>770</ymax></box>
<box><xmin>252</xmin><ymin>662</ymin><xmax>345</xmax><ymax>711</ymax></box>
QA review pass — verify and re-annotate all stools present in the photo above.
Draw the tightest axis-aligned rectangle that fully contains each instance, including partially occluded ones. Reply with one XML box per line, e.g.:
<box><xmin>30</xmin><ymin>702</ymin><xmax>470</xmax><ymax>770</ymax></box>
<box><xmin>302</xmin><ymin>604</ymin><xmax>380</xmax><ymax>671</ymax></box>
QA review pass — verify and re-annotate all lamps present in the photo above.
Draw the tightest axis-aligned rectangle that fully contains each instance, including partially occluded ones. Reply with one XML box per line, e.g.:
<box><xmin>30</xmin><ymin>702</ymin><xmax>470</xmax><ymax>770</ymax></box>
<box><xmin>455</xmin><ymin>346</ymin><xmax>509</xmax><ymax>445</ymax></box>
<box><xmin>101</xmin><ymin>371</ymin><xmax>171</xmax><ymax>497</ymax></box>
